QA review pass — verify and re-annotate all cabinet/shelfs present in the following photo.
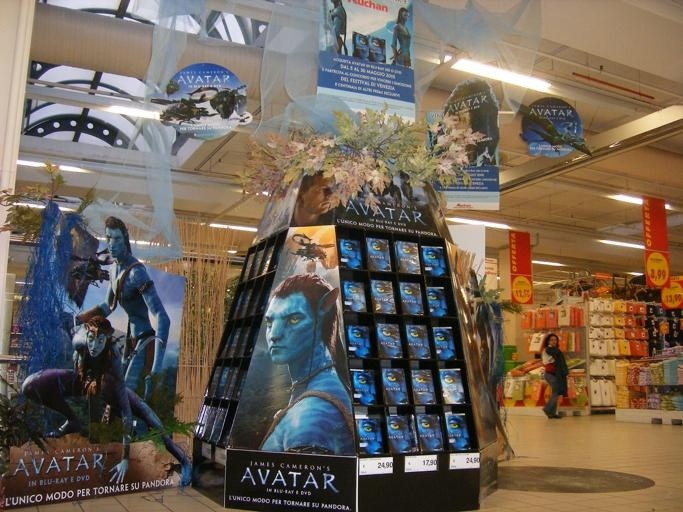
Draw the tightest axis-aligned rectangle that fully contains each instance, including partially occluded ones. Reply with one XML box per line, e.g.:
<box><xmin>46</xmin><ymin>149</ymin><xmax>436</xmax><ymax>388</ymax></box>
<box><xmin>496</xmin><ymin>273</ymin><xmax>683</xmax><ymax>425</ymax></box>
<box><xmin>8</xmin><ymin>282</ymin><xmax>34</xmax><ymax>395</ymax></box>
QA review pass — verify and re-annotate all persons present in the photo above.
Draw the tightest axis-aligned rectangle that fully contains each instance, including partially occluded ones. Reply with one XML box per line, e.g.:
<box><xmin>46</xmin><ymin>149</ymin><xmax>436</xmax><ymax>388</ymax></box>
<box><xmin>352</xmin><ymin>32</ymin><xmax>369</xmax><ymax>61</ymax></box>
<box><xmin>72</xmin><ymin>214</ymin><xmax>171</xmax><ymax>441</ymax></box>
<box><xmin>374</xmin><ymin>165</ymin><xmax>402</xmax><ymax>208</ymax></box>
<box><xmin>538</xmin><ymin>333</ymin><xmax>569</xmax><ymax>420</ymax></box>
<box><xmin>390</xmin><ymin>6</ymin><xmax>411</xmax><ymax>67</ymax></box>
<box><xmin>338</xmin><ymin>237</ymin><xmax>472</xmax><ymax>456</ymax></box>
<box><xmin>290</xmin><ymin>170</ymin><xmax>336</xmax><ymax>226</ymax></box>
<box><xmin>369</xmin><ymin>36</ymin><xmax>388</xmax><ymax>62</ymax></box>
<box><xmin>21</xmin><ymin>316</ymin><xmax>194</xmax><ymax>488</ymax></box>
<box><xmin>255</xmin><ymin>271</ymin><xmax>356</xmax><ymax>456</ymax></box>
<box><xmin>326</xmin><ymin>0</ymin><xmax>348</xmax><ymax>57</ymax></box>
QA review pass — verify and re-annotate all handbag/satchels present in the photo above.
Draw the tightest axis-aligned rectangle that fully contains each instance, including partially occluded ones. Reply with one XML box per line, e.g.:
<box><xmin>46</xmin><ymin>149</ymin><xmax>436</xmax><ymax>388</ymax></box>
<box><xmin>558</xmin><ymin>379</ymin><xmax>566</xmax><ymax>396</ymax></box>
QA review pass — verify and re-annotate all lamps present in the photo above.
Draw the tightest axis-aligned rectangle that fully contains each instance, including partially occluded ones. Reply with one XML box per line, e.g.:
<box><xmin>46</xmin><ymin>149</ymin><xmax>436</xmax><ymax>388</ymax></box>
<box><xmin>571</xmin><ymin>53</ymin><xmax>655</xmax><ymax>101</ymax></box>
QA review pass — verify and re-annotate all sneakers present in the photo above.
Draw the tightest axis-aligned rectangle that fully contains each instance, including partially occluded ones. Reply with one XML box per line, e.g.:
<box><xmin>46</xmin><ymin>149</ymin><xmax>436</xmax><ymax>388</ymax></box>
<box><xmin>543</xmin><ymin>408</ymin><xmax>560</xmax><ymax>418</ymax></box>
